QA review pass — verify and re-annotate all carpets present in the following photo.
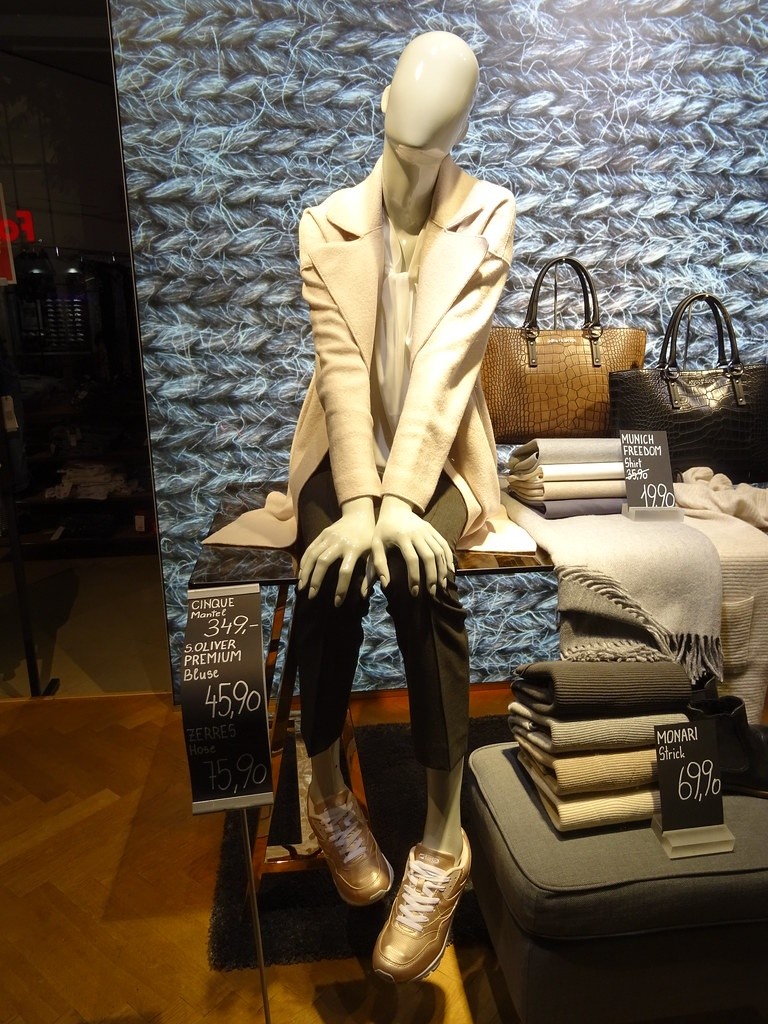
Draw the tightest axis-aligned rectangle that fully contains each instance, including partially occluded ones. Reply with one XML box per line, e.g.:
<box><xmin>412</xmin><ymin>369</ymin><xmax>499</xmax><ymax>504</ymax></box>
<box><xmin>206</xmin><ymin>717</ymin><xmax>513</xmax><ymax>974</ymax></box>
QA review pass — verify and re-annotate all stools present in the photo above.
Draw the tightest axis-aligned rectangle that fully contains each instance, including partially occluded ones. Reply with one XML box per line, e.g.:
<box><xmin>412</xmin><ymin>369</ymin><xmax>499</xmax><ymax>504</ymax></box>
<box><xmin>464</xmin><ymin>741</ymin><xmax>767</xmax><ymax>1024</ymax></box>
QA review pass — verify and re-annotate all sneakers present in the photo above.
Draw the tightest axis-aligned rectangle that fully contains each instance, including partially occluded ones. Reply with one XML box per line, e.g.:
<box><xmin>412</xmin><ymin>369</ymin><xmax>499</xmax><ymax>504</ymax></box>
<box><xmin>373</xmin><ymin>825</ymin><xmax>471</xmax><ymax>984</ymax></box>
<box><xmin>307</xmin><ymin>779</ymin><xmax>394</xmax><ymax>905</ymax></box>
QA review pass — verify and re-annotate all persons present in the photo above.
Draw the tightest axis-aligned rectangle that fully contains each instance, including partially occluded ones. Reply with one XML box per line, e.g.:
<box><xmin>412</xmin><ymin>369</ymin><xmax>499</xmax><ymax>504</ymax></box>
<box><xmin>289</xmin><ymin>30</ymin><xmax>520</xmax><ymax>985</ymax></box>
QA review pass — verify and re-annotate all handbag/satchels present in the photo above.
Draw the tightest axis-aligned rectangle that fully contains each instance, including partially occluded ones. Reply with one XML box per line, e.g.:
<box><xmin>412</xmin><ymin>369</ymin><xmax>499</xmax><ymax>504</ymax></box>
<box><xmin>480</xmin><ymin>255</ymin><xmax>647</xmax><ymax>445</ymax></box>
<box><xmin>608</xmin><ymin>292</ymin><xmax>768</xmax><ymax>486</ymax></box>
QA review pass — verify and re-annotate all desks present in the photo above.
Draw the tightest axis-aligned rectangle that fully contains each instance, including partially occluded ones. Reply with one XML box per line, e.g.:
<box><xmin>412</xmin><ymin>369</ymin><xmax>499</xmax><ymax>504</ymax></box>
<box><xmin>186</xmin><ymin>482</ymin><xmax>552</xmax><ymax>902</ymax></box>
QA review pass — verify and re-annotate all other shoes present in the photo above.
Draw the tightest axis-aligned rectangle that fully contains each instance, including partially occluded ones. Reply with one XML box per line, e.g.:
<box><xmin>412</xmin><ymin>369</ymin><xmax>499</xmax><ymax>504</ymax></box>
<box><xmin>693</xmin><ymin>671</ymin><xmax>768</xmax><ymax>797</ymax></box>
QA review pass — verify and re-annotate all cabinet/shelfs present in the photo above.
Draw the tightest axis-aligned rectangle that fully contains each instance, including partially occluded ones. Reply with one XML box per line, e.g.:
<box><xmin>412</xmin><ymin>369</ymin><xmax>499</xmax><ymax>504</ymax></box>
<box><xmin>0</xmin><ymin>393</ymin><xmax>156</xmax><ymax>549</ymax></box>
